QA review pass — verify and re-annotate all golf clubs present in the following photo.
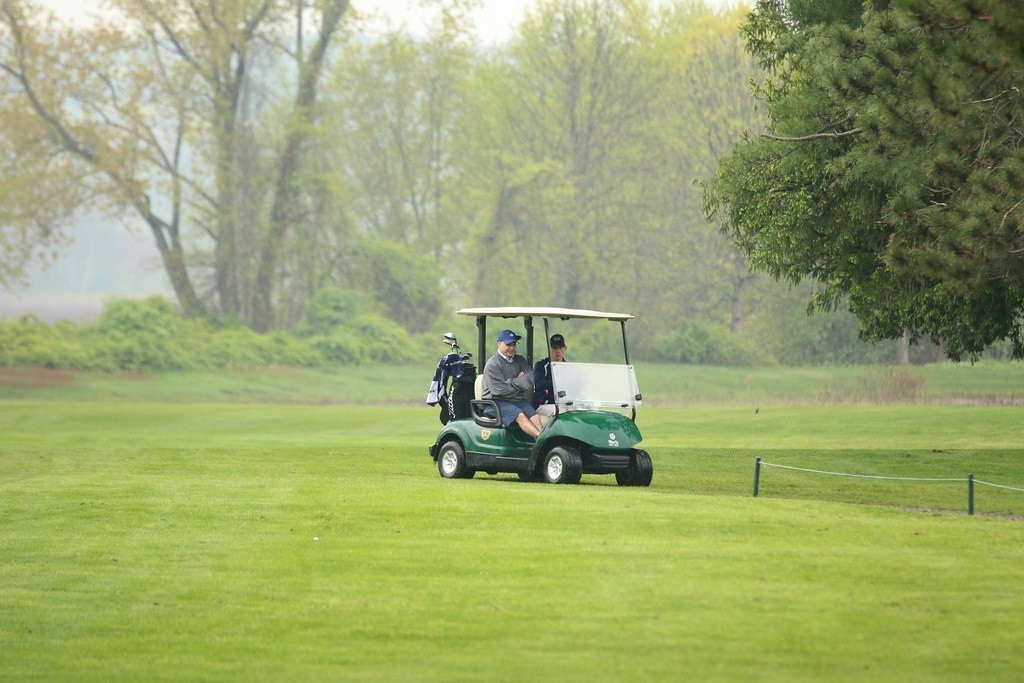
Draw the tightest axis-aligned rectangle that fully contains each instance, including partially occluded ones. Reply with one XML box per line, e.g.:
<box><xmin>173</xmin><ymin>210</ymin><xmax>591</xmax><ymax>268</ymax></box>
<box><xmin>443</xmin><ymin>331</ymin><xmax>473</xmax><ymax>362</ymax></box>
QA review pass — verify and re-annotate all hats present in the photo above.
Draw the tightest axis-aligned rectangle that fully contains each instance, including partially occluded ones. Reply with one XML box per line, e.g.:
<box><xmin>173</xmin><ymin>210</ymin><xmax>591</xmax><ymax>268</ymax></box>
<box><xmin>550</xmin><ymin>334</ymin><xmax>565</xmax><ymax>349</ymax></box>
<box><xmin>497</xmin><ymin>329</ymin><xmax>522</xmax><ymax>345</ymax></box>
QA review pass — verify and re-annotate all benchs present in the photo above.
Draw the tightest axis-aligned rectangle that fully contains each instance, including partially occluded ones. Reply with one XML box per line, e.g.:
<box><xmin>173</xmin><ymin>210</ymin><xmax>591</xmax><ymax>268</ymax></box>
<box><xmin>470</xmin><ymin>374</ymin><xmax>535</xmax><ymax>428</ymax></box>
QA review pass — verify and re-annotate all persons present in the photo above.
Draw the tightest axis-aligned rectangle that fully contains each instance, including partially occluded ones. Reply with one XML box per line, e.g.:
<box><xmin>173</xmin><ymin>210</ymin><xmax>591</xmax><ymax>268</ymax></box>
<box><xmin>534</xmin><ymin>334</ymin><xmax>567</xmax><ymax>416</ymax></box>
<box><xmin>482</xmin><ymin>330</ymin><xmax>543</xmax><ymax>439</ymax></box>
